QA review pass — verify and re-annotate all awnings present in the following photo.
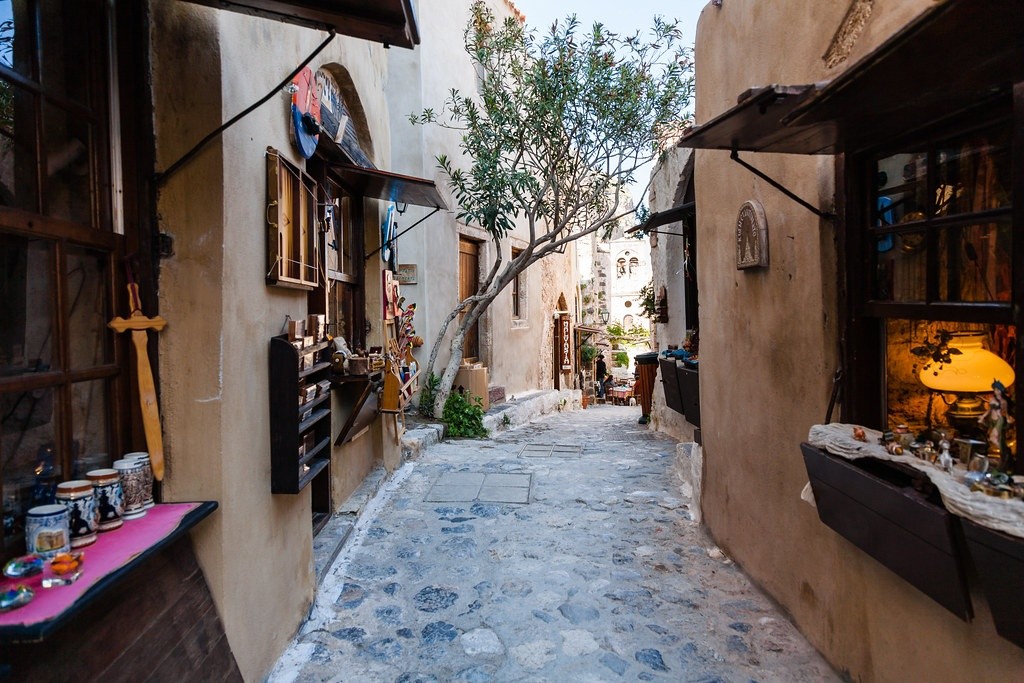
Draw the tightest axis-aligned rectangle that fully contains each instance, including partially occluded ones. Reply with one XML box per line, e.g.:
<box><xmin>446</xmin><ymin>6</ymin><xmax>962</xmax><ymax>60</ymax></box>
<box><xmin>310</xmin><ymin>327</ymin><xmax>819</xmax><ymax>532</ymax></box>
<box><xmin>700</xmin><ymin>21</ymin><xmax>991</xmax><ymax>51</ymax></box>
<box><xmin>623</xmin><ymin>201</ymin><xmax>696</xmax><ymax>234</ymax></box>
<box><xmin>574</xmin><ymin>326</ymin><xmax>601</xmax><ymax>333</ymax></box>
<box><xmin>677</xmin><ymin>0</ymin><xmax>1024</xmax><ymax>156</ymax></box>
<box><xmin>326</xmin><ymin>158</ymin><xmax>450</xmax><ymax>211</ymax></box>
<box><xmin>175</xmin><ymin>0</ymin><xmax>422</xmax><ymax>52</ymax></box>
<box><xmin>596</xmin><ymin>343</ymin><xmax>609</xmax><ymax>347</ymax></box>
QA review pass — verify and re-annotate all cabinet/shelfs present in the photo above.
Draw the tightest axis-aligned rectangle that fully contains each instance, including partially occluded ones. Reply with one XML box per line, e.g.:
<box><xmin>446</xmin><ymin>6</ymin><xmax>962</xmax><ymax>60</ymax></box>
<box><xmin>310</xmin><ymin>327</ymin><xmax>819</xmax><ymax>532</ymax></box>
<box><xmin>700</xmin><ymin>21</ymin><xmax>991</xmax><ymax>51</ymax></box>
<box><xmin>269</xmin><ymin>332</ymin><xmax>332</xmax><ymax>495</ymax></box>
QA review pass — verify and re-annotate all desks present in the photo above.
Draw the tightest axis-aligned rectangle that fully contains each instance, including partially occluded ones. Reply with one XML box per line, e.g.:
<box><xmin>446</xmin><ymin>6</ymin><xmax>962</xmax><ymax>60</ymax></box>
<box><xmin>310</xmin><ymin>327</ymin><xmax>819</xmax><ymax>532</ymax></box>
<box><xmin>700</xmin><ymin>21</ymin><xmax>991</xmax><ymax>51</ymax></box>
<box><xmin>613</xmin><ymin>386</ymin><xmax>632</xmax><ymax>406</ymax></box>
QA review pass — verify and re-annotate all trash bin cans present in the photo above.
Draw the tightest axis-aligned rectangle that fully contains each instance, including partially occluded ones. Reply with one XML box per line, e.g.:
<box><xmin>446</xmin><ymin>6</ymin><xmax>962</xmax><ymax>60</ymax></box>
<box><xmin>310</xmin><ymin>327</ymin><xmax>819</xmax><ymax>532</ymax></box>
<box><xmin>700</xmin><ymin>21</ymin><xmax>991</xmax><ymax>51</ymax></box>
<box><xmin>635</xmin><ymin>353</ymin><xmax>659</xmax><ymax>424</ymax></box>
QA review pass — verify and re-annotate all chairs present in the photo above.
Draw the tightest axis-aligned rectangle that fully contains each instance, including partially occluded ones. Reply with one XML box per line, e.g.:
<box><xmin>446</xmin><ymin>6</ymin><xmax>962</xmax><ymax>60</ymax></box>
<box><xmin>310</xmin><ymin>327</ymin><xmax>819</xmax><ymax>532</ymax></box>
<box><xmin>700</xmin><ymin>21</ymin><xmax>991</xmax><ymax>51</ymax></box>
<box><xmin>605</xmin><ymin>384</ymin><xmax>619</xmax><ymax>405</ymax></box>
<box><xmin>631</xmin><ymin>386</ymin><xmax>641</xmax><ymax>406</ymax></box>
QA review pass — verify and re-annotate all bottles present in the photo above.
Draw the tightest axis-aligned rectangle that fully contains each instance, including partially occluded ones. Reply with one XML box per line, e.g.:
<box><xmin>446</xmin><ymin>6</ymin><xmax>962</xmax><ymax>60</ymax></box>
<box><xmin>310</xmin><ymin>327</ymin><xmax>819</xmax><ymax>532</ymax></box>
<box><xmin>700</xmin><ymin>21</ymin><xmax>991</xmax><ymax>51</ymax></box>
<box><xmin>54</xmin><ymin>451</ymin><xmax>155</xmax><ymax>548</ymax></box>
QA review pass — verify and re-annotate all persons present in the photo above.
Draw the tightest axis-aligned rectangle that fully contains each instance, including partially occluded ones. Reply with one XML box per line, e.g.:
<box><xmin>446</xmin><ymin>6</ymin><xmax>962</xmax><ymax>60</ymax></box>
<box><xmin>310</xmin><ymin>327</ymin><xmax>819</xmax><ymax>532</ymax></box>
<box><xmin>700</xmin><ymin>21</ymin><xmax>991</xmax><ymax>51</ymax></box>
<box><xmin>595</xmin><ymin>355</ymin><xmax>607</xmax><ymax>397</ymax></box>
<box><xmin>632</xmin><ymin>362</ymin><xmax>642</xmax><ymax>395</ymax></box>
<box><xmin>603</xmin><ymin>372</ymin><xmax>618</xmax><ymax>393</ymax></box>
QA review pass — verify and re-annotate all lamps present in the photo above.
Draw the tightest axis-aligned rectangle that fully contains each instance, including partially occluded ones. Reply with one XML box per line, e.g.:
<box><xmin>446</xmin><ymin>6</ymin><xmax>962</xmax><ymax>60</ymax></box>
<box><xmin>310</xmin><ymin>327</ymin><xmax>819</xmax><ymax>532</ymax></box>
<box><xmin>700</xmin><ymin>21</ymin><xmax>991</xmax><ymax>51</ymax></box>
<box><xmin>594</xmin><ymin>307</ymin><xmax>609</xmax><ymax>325</ymax></box>
<box><xmin>918</xmin><ymin>324</ymin><xmax>1016</xmax><ymax>443</ymax></box>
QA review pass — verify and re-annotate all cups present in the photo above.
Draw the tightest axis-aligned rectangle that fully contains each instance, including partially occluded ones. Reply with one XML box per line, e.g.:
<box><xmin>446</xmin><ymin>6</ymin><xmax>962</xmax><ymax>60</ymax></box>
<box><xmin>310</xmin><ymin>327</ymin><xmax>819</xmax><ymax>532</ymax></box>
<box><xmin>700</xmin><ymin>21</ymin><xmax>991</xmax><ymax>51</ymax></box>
<box><xmin>25</xmin><ymin>504</ymin><xmax>72</xmax><ymax>561</ymax></box>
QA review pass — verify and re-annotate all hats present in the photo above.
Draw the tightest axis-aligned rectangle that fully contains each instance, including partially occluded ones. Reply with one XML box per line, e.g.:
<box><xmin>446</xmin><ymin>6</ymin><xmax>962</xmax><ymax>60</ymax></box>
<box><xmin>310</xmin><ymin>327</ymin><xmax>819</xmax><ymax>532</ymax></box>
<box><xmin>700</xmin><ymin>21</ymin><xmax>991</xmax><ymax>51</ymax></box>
<box><xmin>598</xmin><ymin>355</ymin><xmax>604</xmax><ymax>359</ymax></box>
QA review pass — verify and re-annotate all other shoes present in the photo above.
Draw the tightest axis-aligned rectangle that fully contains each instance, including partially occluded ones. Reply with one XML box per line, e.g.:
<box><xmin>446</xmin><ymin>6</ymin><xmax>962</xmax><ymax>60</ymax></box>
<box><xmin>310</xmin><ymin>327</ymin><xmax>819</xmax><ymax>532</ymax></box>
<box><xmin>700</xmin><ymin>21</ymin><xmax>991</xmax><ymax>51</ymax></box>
<box><xmin>597</xmin><ymin>395</ymin><xmax>603</xmax><ymax>398</ymax></box>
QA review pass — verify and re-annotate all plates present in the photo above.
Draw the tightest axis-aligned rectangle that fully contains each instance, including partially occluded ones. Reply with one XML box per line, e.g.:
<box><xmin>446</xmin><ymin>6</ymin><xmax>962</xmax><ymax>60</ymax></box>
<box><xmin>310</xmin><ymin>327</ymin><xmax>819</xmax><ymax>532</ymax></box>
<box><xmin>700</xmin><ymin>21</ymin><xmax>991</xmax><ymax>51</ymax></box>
<box><xmin>0</xmin><ymin>582</ymin><xmax>35</xmax><ymax>612</ymax></box>
<box><xmin>2</xmin><ymin>552</ymin><xmax>52</xmax><ymax>577</ymax></box>
